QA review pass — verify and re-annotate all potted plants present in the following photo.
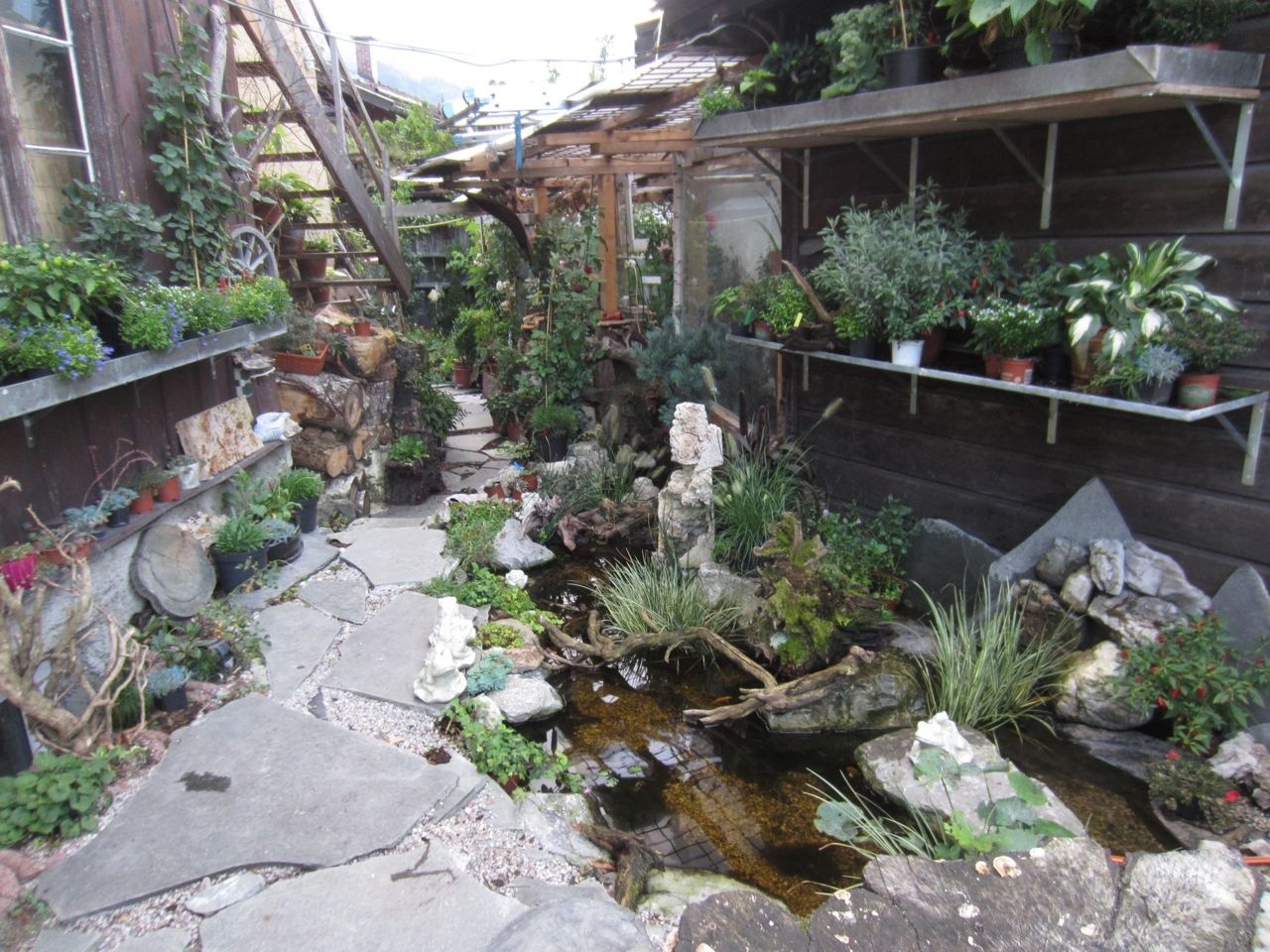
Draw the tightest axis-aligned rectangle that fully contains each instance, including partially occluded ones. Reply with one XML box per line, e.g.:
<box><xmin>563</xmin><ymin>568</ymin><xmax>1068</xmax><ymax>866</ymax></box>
<box><xmin>698</xmin><ymin>0</ymin><xmax>1254</xmax><ymax>119</ymax></box>
<box><xmin>709</xmin><ymin>178</ymin><xmax>1259</xmax><ymax>407</ymax></box>
<box><xmin>388</xmin><ymin>195</ymin><xmax>672</xmax><ymax>502</ymax></box>
<box><xmin>207</xmin><ymin>461</ymin><xmax>326</xmax><ymax>593</ymax></box>
<box><xmin>111</xmin><ymin>663</ymin><xmax>189</xmax><ymax>732</ymax></box>
<box><xmin>0</xmin><ymin>457</ymin><xmax>200</xmax><ymax>591</ymax></box>
<box><xmin>255</xmin><ymin>172</ymin><xmax>372</xmax><ymax>376</ymax></box>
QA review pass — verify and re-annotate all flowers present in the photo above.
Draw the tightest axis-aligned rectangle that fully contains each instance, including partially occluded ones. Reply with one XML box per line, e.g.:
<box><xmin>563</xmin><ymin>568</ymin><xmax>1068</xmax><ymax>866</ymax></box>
<box><xmin>966</xmin><ymin>298</ymin><xmax>1060</xmax><ymax>358</ymax></box>
<box><xmin>820</xmin><ymin>494</ymin><xmax>918</xmax><ymax>619</ymax></box>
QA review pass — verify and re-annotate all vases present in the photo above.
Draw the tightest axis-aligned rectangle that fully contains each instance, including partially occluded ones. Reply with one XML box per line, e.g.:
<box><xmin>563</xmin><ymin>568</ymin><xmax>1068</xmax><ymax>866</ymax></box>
<box><xmin>847</xmin><ymin>575</ymin><xmax>907</xmax><ymax>612</ymax></box>
<box><xmin>995</xmin><ymin>357</ymin><xmax>1036</xmax><ymax>385</ymax></box>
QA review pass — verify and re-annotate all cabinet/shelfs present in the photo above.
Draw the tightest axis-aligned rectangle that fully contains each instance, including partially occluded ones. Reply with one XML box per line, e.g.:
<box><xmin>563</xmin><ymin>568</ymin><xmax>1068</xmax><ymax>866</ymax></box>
<box><xmin>0</xmin><ymin>313</ymin><xmax>292</xmax><ymax>595</ymax></box>
<box><xmin>696</xmin><ymin>46</ymin><xmax>1270</xmax><ymax>485</ymax></box>
